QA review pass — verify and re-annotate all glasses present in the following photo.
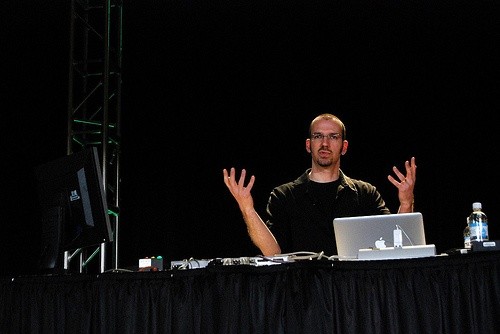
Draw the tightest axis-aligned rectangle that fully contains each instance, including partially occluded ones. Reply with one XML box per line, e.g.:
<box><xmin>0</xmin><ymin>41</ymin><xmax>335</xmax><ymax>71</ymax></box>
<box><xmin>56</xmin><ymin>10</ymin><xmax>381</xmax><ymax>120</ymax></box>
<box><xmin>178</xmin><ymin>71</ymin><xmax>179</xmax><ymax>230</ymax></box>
<box><xmin>310</xmin><ymin>132</ymin><xmax>342</xmax><ymax>141</ymax></box>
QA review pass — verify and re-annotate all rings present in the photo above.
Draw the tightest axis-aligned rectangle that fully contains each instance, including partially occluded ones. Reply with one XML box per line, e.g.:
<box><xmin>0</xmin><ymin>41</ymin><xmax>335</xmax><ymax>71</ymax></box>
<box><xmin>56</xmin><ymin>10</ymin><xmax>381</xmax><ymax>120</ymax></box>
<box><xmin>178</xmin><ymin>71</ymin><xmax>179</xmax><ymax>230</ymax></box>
<box><xmin>401</xmin><ymin>178</ymin><xmax>407</xmax><ymax>183</ymax></box>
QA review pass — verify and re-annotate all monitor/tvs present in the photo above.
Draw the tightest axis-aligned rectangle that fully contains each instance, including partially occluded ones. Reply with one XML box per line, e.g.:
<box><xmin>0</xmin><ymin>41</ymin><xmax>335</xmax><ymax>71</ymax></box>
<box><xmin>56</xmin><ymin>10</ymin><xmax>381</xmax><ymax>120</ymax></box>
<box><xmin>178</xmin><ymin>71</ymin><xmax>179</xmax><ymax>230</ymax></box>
<box><xmin>36</xmin><ymin>147</ymin><xmax>113</xmax><ymax>277</ymax></box>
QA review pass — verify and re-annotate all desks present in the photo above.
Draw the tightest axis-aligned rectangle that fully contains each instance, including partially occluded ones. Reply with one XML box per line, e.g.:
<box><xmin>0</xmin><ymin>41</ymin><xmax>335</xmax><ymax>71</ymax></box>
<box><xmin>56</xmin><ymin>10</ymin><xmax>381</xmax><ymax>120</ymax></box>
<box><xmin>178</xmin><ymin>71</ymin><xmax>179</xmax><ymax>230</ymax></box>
<box><xmin>0</xmin><ymin>250</ymin><xmax>500</xmax><ymax>334</ymax></box>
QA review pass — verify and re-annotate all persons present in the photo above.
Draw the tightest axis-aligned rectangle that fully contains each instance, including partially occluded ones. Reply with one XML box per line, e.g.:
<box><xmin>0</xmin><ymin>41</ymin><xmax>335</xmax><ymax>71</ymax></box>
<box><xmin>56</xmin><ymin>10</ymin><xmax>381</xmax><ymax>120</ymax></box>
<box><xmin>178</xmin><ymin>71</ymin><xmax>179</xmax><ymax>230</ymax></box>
<box><xmin>223</xmin><ymin>113</ymin><xmax>417</xmax><ymax>257</ymax></box>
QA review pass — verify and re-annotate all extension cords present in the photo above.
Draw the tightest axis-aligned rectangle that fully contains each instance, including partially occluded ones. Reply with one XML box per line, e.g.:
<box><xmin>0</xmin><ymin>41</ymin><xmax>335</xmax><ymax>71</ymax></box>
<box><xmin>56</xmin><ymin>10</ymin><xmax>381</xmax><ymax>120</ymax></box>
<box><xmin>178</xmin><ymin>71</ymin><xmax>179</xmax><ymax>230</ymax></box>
<box><xmin>358</xmin><ymin>244</ymin><xmax>436</xmax><ymax>260</ymax></box>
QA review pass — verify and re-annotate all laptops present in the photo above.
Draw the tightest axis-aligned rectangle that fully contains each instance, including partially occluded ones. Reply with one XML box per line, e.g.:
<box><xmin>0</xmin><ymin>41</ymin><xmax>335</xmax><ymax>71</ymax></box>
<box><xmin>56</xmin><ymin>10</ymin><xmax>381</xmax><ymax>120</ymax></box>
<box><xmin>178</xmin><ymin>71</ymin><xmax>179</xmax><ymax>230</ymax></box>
<box><xmin>333</xmin><ymin>212</ymin><xmax>426</xmax><ymax>261</ymax></box>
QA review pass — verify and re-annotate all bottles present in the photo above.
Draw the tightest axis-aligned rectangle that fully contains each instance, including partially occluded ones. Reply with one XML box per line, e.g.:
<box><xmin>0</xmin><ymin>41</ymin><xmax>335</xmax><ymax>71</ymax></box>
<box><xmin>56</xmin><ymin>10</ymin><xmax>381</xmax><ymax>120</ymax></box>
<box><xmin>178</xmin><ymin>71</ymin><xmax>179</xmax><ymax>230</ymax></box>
<box><xmin>469</xmin><ymin>203</ymin><xmax>489</xmax><ymax>246</ymax></box>
<box><xmin>463</xmin><ymin>217</ymin><xmax>472</xmax><ymax>249</ymax></box>
